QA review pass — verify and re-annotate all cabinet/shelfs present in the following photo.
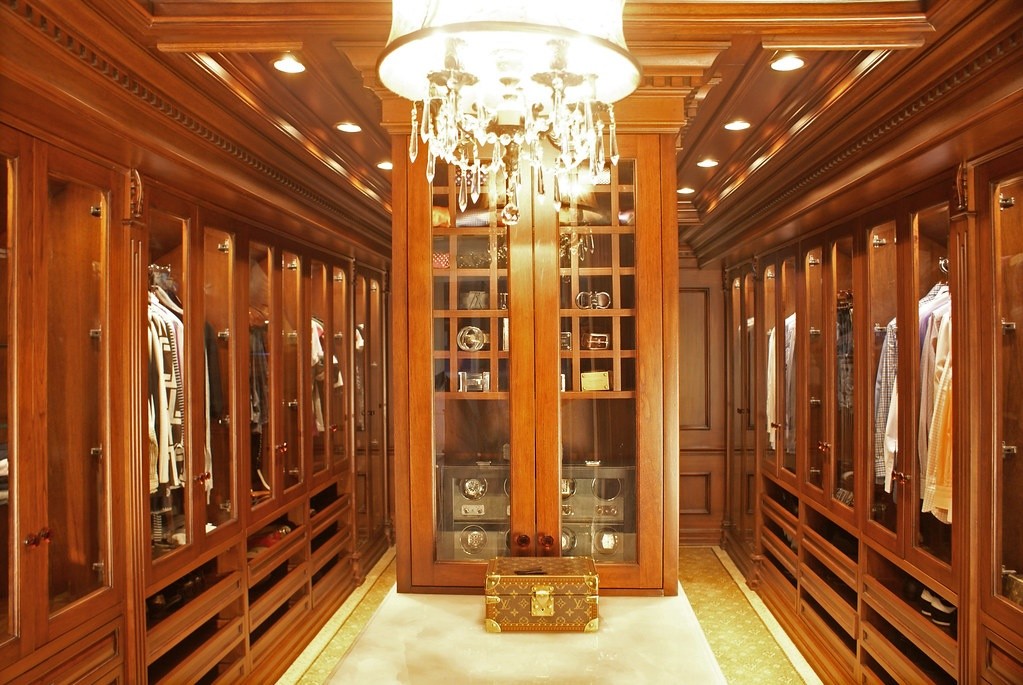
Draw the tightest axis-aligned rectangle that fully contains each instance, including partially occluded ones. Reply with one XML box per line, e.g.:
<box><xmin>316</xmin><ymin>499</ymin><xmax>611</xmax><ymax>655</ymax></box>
<box><xmin>361</xmin><ymin>72</ymin><xmax>701</xmax><ymax>597</ymax></box>
<box><xmin>721</xmin><ymin>0</ymin><xmax>1023</xmax><ymax>685</ymax></box>
<box><xmin>1</xmin><ymin>3</ymin><xmax>398</xmax><ymax>685</ymax></box>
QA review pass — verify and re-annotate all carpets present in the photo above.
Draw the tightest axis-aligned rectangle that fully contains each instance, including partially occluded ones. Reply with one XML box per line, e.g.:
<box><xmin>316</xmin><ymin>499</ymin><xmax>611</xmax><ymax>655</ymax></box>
<box><xmin>321</xmin><ymin>581</ymin><xmax>728</xmax><ymax>685</ymax></box>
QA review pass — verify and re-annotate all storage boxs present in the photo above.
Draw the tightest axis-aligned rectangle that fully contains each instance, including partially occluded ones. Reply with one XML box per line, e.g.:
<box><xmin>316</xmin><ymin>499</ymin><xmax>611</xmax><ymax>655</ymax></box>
<box><xmin>483</xmin><ymin>557</ymin><xmax>599</xmax><ymax>634</ymax></box>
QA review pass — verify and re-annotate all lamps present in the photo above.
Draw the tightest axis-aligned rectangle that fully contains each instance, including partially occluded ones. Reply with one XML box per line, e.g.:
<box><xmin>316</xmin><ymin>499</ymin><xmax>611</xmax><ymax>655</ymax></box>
<box><xmin>376</xmin><ymin>0</ymin><xmax>642</xmax><ymax>223</ymax></box>
